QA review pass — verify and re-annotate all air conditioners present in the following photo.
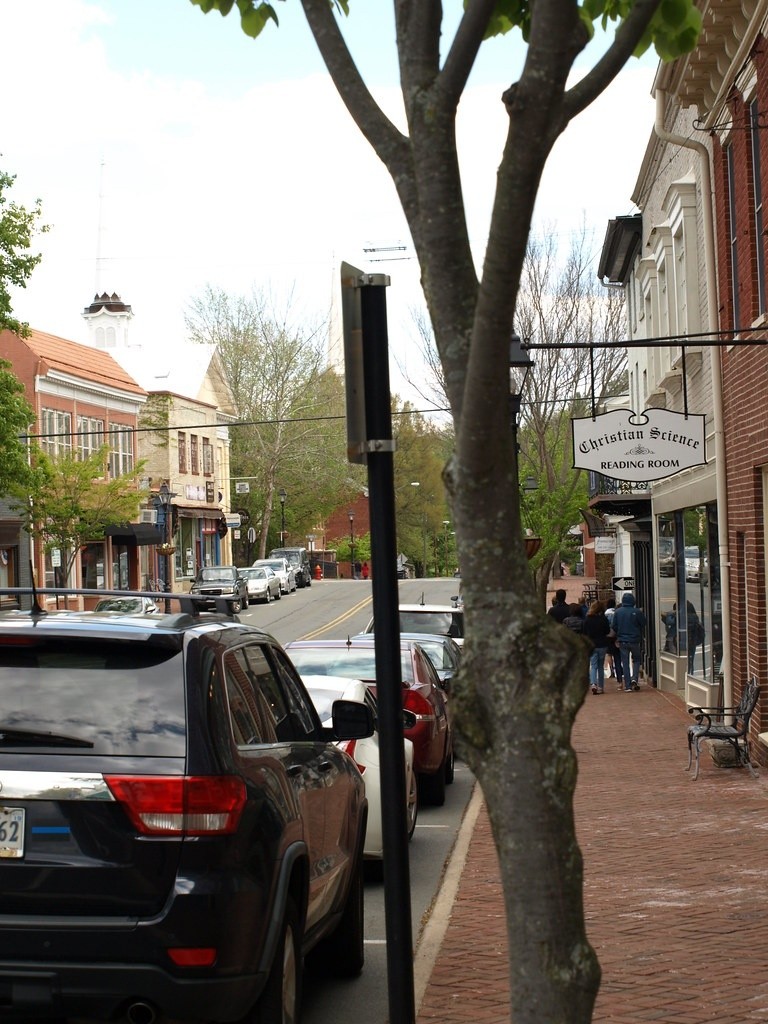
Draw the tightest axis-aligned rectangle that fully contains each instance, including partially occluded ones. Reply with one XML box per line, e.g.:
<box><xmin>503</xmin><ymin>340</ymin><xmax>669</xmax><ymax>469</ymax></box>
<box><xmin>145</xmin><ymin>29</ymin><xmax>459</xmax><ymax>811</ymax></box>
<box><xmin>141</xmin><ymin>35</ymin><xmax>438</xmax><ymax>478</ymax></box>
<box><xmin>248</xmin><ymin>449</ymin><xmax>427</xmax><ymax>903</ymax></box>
<box><xmin>139</xmin><ymin>509</ymin><xmax>158</xmax><ymax>524</ymax></box>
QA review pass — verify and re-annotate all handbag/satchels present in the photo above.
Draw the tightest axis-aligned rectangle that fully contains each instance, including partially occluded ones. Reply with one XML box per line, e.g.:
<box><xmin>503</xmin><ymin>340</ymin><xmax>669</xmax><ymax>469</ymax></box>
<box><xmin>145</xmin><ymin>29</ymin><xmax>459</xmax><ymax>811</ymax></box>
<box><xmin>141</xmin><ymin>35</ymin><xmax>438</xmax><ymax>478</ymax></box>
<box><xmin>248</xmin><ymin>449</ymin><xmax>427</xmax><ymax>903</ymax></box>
<box><xmin>694</xmin><ymin>623</ymin><xmax>704</xmax><ymax>646</ymax></box>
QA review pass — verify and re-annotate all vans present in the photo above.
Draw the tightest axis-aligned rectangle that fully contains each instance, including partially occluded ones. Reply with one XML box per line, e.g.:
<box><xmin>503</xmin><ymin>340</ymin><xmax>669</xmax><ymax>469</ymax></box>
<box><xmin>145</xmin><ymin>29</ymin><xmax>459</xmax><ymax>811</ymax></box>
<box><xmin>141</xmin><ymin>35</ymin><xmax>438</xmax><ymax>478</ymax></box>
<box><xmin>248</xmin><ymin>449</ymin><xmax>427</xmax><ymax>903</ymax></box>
<box><xmin>268</xmin><ymin>547</ymin><xmax>312</xmax><ymax>588</ymax></box>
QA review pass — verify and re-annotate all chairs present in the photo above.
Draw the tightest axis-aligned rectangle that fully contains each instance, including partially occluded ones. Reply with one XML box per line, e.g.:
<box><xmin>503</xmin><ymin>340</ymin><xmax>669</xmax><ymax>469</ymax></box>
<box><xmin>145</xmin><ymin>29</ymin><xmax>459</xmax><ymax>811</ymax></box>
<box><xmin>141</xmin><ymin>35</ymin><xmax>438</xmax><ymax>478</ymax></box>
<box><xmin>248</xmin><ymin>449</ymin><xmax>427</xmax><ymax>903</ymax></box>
<box><xmin>683</xmin><ymin>677</ymin><xmax>762</xmax><ymax>781</ymax></box>
<box><xmin>296</xmin><ymin>649</ymin><xmax>328</xmax><ymax>675</ymax></box>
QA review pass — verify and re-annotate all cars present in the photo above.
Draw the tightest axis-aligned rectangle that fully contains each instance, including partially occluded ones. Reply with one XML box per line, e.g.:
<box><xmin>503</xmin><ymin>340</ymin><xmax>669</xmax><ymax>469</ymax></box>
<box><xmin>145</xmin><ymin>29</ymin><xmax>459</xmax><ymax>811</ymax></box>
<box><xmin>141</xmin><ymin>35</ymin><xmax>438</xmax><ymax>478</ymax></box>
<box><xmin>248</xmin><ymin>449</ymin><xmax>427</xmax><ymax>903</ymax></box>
<box><xmin>94</xmin><ymin>596</ymin><xmax>159</xmax><ymax>615</ymax></box>
<box><xmin>237</xmin><ymin>558</ymin><xmax>297</xmax><ymax>602</ymax></box>
<box><xmin>96</xmin><ymin>552</ymin><xmax>128</xmax><ymax>589</ymax></box>
<box><xmin>299</xmin><ymin>675</ymin><xmax>417</xmax><ymax>861</ymax></box>
<box><xmin>659</xmin><ymin>536</ymin><xmax>708</xmax><ymax>583</ymax></box>
<box><xmin>284</xmin><ymin>603</ymin><xmax>467</xmax><ymax>806</ymax></box>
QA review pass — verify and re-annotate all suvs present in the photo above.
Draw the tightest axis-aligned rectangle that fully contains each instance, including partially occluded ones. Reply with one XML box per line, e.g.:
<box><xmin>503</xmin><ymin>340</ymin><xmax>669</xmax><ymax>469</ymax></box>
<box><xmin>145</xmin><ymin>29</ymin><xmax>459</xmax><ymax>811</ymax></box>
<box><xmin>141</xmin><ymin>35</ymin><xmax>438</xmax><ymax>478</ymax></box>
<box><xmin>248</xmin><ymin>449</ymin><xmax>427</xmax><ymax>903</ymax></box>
<box><xmin>189</xmin><ymin>566</ymin><xmax>249</xmax><ymax>613</ymax></box>
<box><xmin>0</xmin><ymin>609</ymin><xmax>368</xmax><ymax>1024</ymax></box>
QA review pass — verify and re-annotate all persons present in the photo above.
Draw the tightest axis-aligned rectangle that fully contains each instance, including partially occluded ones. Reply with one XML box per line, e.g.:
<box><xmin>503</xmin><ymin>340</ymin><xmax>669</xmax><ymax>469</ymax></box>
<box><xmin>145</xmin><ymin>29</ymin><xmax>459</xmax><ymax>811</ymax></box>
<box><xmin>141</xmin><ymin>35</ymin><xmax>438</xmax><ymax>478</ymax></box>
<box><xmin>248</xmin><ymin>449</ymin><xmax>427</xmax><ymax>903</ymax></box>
<box><xmin>661</xmin><ymin>601</ymin><xmax>699</xmax><ymax>675</ymax></box>
<box><xmin>548</xmin><ymin>589</ymin><xmax>570</xmax><ymax>623</ymax></box>
<box><xmin>578</xmin><ymin>596</ymin><xmax>588</xmax><ymax>617</ymax></box>
<box><xmin>612</xmin><ymin>593</ymin><xmax>645</xmax><ymax>692</ymax></box>
<box><xmin>616</xmin><ymin>603</ymin><xmax>623</xmax><ymax>691</ymax></box>
<box><xmin>605</xmin><ymin>599</ymin><xmax>616</xmax><ymax>678</ymax></box>
<box><xmin>453</xmin><ymin>567</ymin><xmax>460</xmax><ymax>577</ymax></box>
<box><xmin>563</xmin><ymin>603</ymin><xmax>583</xmax><ymax>633</ymax></box>
<box><xmin>583</xmin><ymin>601</ymin><xmax>611</xmax><ymax>695</ymax></box>
<box><xmin>362</xmin><ymin>562</ymin><xmax>369</xmax><ymax>580</ymax></box>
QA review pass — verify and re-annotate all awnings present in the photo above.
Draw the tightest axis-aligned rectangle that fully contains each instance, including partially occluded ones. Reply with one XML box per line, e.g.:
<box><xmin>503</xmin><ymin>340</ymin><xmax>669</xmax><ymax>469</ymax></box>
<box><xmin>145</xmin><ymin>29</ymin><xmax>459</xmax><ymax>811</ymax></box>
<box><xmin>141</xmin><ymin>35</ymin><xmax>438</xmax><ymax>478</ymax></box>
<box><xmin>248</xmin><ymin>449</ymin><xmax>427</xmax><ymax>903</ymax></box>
<box><xmin>106</xmin><ymin>524</ymin><xmax>162</xmax><ymax>546</ymax></box>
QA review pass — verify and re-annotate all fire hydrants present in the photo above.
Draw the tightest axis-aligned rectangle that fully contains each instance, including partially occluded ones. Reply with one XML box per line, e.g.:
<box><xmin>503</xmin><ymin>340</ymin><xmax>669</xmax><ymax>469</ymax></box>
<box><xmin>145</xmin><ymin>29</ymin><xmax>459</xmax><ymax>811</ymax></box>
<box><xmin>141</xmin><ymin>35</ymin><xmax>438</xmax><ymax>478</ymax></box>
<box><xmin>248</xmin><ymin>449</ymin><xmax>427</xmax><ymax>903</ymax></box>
<box><xmin>315</xmin><ymin>565</ymin><xmax>322</xmax><ymax>580</ymax></box>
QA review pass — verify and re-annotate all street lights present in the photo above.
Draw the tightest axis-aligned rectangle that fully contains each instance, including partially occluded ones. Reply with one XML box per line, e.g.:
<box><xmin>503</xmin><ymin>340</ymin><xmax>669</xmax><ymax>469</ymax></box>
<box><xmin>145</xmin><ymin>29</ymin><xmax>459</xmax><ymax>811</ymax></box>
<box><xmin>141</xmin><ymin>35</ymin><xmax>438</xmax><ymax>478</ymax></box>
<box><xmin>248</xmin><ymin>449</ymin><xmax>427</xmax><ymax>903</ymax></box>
<box><xmin>347</xmin><ymin>507</ymin><xmax>356</xmax><ymax>579</ymax></box>
<box><xmin>277</xmin><ymin>487</ymin><xmax>287</xmax><ymax>547</ymax></box>
<box><xmin>158</xmin><ymin>481</ymin><xmax>172</xmax><ymax>613</ymax></box>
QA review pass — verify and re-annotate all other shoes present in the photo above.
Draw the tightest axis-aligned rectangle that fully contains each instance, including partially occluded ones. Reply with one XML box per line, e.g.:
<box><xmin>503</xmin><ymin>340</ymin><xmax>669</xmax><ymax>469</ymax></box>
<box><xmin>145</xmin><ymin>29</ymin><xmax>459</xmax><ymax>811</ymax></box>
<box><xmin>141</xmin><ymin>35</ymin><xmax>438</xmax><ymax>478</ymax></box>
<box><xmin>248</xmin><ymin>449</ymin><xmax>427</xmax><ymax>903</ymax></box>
<box><xmin>592</xmin><ymin>684</ymin><xmax>603</xmax><ymax>694</ymax></box>
<box><xmin>617</xmin><ymin>684</ymin><xmax>623</xmax><ymax>692</ymax></box>
<box><xmin>608</xmin><ymin>675</ymin><xmax>616</xmax><ymax>679</ymax></box>
<box><xmin>631</xmin><ymin>680</ymin><xmax>640</xmax><ymax>692</ymax></box>
<box><xmin>624</xmin><ymin>687</ymin><xmax>631</xmax><ymax>692</ymax></box>
